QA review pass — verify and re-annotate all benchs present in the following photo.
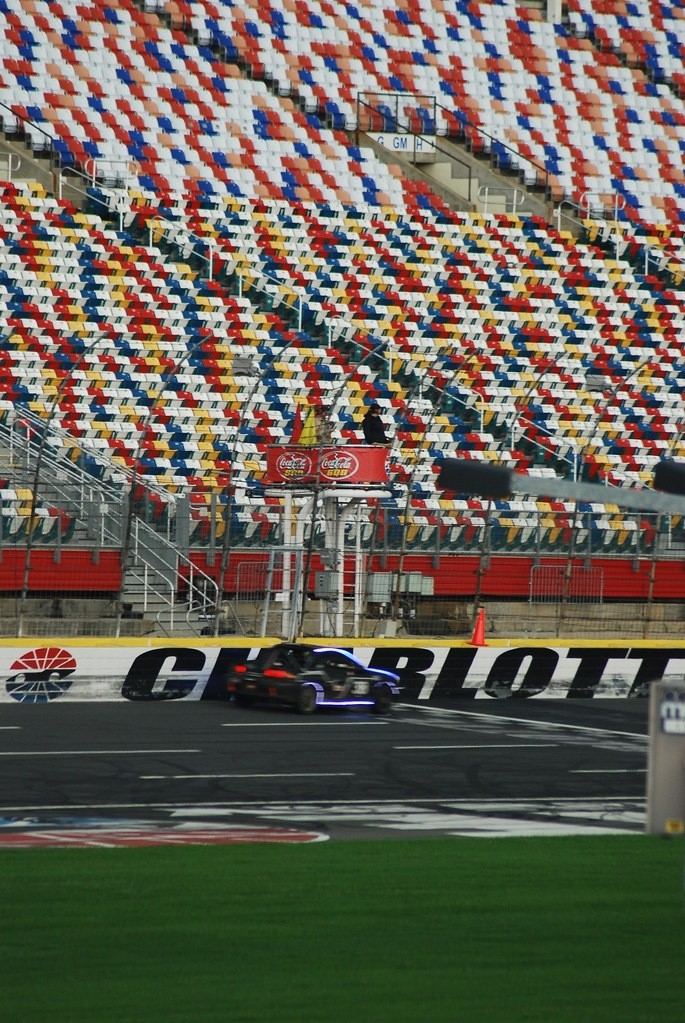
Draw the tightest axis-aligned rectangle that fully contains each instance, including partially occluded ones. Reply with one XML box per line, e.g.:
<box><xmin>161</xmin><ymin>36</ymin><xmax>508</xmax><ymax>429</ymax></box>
<box><xmin>2</xmin><ymin>0</ymin><xmax>685</xmax><ymax>552</ymax></box>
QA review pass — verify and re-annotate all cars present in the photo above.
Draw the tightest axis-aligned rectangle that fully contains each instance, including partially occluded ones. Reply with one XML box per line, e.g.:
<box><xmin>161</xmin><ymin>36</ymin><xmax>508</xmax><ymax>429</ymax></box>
<box><xmin>226</xmin><ymin>642</ymin><xmax>400</xmax><ymax>716</ymax></box>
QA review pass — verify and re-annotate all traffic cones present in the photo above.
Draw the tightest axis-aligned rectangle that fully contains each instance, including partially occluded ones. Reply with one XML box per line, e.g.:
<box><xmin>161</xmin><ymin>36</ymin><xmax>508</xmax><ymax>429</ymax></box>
<box><xmin>466</xmin><ymin>609</ymin><xmax>492</xmax><ymax>647</ymax></box>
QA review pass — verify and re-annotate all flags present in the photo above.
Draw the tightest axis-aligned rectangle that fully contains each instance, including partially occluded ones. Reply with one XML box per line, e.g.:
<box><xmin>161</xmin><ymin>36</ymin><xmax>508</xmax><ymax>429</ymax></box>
<box><xmin>265</xmin><ymin>447</ymin><xmax>391</xmax><ymax>483</ymax></box>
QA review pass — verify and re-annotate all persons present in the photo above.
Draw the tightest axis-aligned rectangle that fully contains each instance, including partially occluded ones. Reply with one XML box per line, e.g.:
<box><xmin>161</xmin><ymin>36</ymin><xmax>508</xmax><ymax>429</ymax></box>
<box><xmin>361</xmin><ymin>402</ymin><xmax>393</xmax><ymax>489</ymax></box>
<box><xmin>314</xmin><ymin>405</ymin><xmax>336</xmax><ymax>444</ymax></box>
